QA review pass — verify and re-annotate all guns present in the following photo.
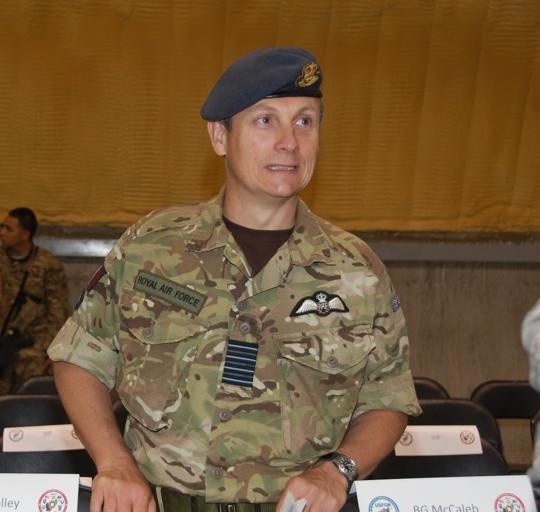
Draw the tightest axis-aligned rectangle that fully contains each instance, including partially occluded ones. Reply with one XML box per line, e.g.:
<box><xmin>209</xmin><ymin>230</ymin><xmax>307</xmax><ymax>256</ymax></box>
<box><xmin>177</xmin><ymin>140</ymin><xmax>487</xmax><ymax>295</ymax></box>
<box><xmin>0</xmin><ymin>270</ymin><xmax>43</xmax><ymax>372</ymax></box>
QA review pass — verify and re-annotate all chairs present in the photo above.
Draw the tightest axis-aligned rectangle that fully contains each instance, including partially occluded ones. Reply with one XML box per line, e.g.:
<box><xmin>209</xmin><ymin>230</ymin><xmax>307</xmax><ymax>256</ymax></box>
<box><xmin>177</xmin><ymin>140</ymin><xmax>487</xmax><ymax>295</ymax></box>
<box><xmin>0</xmin><ymin>374</ymin><xmax>540</xmax><ymax>511</ymax></box>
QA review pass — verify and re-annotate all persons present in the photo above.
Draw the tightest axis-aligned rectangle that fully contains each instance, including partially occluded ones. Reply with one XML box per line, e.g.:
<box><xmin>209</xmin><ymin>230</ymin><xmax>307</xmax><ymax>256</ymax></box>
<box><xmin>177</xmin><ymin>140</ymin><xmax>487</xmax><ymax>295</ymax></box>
<box><xmin>49</xmin><ymin>43</ymin><xmax>424</xmax><ymax>512</ymax></box>
<box><xmin>0</xmin><ymin>207</ymin><xmax>71</xmax><ymax>400</ymax></box>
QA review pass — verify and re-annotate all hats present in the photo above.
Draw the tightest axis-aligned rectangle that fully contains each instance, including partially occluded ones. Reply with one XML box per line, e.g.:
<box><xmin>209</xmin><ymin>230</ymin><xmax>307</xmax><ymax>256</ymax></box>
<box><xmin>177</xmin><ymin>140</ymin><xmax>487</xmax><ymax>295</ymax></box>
<box><xmin>199</xmin><ymin>45</ymin><xmax>324</xmax><ymax>123</ymax></box>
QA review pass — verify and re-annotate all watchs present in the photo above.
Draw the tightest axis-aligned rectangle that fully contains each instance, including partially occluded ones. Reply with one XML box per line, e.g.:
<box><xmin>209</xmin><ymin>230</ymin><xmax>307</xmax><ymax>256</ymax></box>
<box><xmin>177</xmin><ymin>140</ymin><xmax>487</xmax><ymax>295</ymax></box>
<box><xmin>312</xmin><ymin>451</ymin><xmax>360</xmax><ymax>494</ymax></box>
